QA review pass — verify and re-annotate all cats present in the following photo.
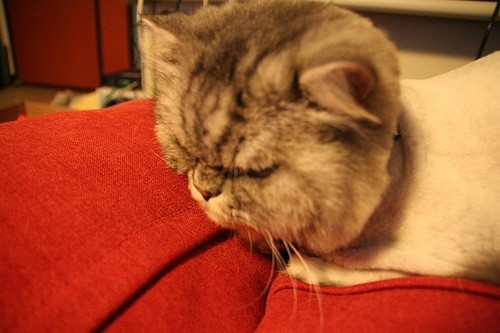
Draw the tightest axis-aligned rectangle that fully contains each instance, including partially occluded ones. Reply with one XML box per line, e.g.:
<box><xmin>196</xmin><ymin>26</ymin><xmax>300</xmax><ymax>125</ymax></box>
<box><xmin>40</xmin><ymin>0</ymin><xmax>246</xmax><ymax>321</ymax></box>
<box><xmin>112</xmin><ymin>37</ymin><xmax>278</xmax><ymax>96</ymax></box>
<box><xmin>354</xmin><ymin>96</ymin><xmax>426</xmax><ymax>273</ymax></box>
<box><xmin>132</xmin><ymin>0</ymin><xmax>500</xmax><ymax>288</ymax></box>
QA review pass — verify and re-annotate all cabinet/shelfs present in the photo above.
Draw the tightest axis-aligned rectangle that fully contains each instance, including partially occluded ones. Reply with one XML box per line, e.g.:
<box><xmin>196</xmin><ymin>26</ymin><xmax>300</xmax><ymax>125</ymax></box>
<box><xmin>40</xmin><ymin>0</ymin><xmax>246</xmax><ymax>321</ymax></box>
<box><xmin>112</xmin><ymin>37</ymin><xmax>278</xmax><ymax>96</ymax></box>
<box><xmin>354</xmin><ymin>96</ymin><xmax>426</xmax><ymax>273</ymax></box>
<box><xmin>0</xmin><ymin>0</ymin><xmax>139</xmax><ymax>92</ymax></box>
